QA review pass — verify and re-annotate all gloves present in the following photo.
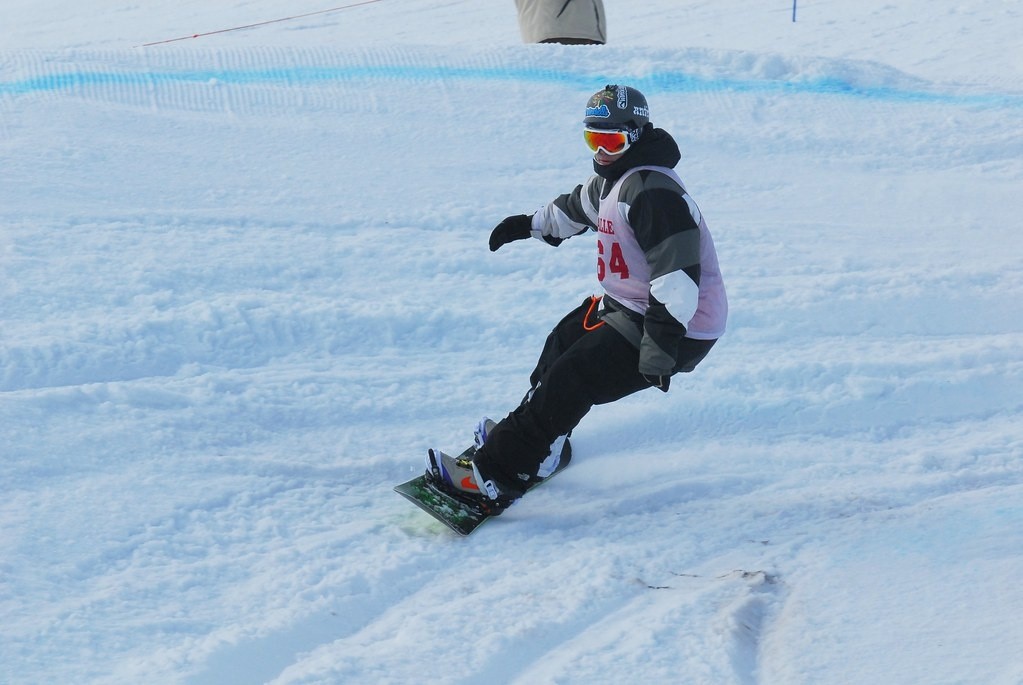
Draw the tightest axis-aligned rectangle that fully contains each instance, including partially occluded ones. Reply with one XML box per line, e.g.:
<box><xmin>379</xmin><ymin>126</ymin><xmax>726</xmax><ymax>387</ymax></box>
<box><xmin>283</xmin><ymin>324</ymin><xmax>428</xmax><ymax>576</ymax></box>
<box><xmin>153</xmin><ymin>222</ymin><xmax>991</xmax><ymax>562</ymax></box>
<box><xmin>489</xmin><ymin>214</ymin><xmax>533</xmax><ymax>251</ymax></box>
<box><xmin>642</xmin><ymin>373</ymin><xmax>670</xmax><ymax>393</ymax></box>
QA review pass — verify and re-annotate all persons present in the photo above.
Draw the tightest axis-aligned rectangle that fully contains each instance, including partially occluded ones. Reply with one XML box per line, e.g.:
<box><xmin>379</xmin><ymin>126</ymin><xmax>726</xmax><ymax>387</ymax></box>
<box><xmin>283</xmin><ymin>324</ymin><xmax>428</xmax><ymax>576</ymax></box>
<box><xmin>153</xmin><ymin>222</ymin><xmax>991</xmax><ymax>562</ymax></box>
<box><xmin>515</xmin><ymin>0</ymin><xmax>606</xmax><ymax>46</ymax></box>
<box><xmin>423</xmin><ymin>83</ymin><xmax>730</xmax><ymax>518</ymax></box>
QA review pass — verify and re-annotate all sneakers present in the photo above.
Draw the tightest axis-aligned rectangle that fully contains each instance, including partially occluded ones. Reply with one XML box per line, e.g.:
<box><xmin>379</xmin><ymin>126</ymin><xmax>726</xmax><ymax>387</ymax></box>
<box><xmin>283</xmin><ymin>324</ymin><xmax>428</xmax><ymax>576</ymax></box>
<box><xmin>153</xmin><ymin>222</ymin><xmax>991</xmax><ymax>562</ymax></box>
<box><xmin>473</xmin><ymin>417</ymin><xmax>549</xmax><ymax>481</ymax></box>
<box><xmin>424</xmin><ymin>449</ymin><xmax>513</xmax><ymax>517</ymax></box>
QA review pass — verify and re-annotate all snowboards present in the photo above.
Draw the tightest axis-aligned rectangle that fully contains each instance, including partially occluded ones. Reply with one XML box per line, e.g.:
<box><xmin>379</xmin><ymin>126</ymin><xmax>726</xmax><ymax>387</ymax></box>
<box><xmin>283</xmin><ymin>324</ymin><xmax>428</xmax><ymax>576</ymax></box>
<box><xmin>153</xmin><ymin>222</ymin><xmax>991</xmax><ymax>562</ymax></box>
<box><xmin>392</xmin><ymin>417</ymin><xmax>575</xmax><ymax>540</ymax></box>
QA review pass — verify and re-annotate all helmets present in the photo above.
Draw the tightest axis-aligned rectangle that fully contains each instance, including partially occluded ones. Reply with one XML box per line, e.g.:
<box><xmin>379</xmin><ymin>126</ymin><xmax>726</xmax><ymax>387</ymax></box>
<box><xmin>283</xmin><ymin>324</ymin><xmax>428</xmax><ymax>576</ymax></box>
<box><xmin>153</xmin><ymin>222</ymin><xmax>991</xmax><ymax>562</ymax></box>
<box><xmin>585</xmin><ymin>85</ymin><xmax>648</xmax><ymax>138</ymax></box>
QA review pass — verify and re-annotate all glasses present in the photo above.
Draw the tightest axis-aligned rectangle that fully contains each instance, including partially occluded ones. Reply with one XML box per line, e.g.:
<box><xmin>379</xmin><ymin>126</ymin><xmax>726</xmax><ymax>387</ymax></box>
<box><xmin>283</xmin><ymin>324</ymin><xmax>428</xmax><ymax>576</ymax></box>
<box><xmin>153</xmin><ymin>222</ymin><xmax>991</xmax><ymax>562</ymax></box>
<box><xmin>583</xmin><ymin>128</ymin><xmax>631</xmax><ymax>155</ymax></box>
